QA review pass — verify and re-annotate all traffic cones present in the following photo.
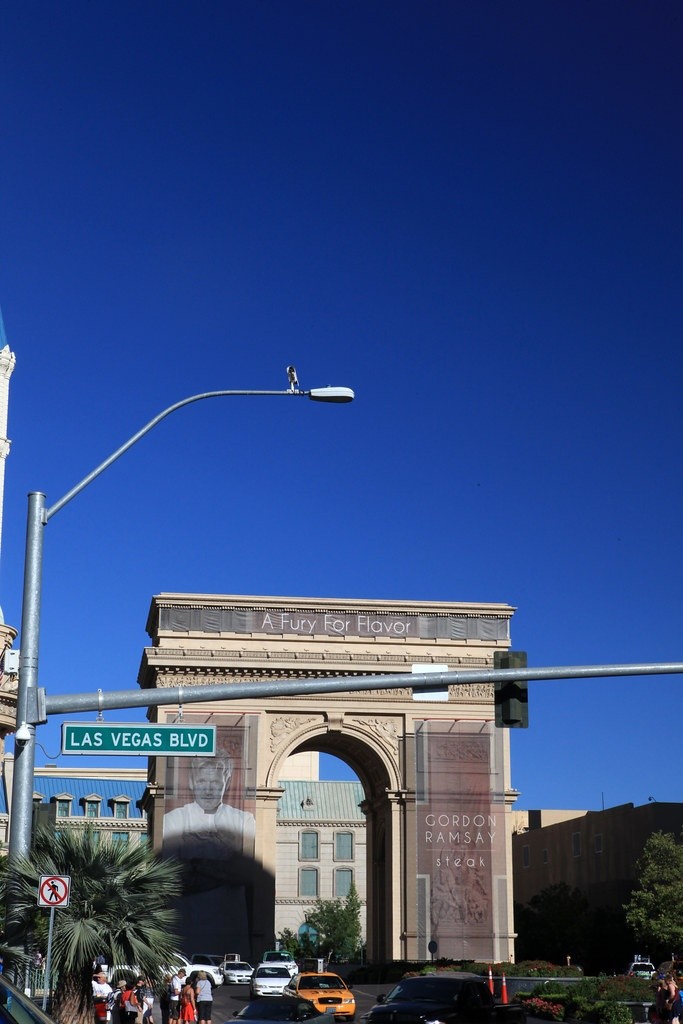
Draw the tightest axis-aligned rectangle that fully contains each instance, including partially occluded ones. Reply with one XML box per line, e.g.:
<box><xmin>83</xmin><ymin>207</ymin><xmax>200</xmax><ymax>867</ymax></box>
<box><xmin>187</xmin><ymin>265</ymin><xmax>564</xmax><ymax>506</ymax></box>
<box><xmin>499</xmin><ymin>971</ymin><xmax>509</xmax><ymax>1005</ymax></box>
<box><xmin>487</xmin><ymin>966</ymin><xmax>495</xmax><ymax>995</ymax></box>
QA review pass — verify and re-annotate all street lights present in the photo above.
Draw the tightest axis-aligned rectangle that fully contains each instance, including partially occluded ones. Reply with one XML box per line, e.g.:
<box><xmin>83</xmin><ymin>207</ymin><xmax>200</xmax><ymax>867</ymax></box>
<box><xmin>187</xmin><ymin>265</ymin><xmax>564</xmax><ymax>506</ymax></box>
<box><xmin>5</xmin><ymin>367</ymin><xmax>356</xmax><ymax>942</ymax></box>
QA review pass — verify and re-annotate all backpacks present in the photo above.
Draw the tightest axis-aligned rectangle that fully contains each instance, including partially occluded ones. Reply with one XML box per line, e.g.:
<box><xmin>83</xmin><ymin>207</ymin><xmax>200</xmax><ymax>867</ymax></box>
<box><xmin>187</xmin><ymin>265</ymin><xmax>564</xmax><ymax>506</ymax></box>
<box><xmin>104</xmin><ymin>990</ymin><xmax>121</xmax><ymax>1010</ymax></box>
<box><xmin>181</xmin><ymin>987</ymin><xmax>192</xmax><ymax>1006</ymax></box>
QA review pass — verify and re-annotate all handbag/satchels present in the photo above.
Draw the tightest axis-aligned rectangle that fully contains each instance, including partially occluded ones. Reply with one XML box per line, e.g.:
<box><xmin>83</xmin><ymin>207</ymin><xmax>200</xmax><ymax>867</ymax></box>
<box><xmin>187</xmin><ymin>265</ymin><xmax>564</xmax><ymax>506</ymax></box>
<box><xmin>124</xmin><ymin>1000</ymin><xmax>138</xmax><ymax>1015</ymax></box>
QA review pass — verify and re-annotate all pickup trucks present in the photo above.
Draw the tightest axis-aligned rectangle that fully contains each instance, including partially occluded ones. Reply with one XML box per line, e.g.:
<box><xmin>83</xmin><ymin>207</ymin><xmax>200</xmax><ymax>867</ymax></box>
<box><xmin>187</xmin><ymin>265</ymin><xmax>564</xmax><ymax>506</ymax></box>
<box><xmin>360</xmin><ymin>971</ymin><xmax>528</xmax><ymax>1024</ymax></box>
<box><xmin>625</xmin><ymin>961</ymin><xmax>657</xmax><ymax>979</ymax></box>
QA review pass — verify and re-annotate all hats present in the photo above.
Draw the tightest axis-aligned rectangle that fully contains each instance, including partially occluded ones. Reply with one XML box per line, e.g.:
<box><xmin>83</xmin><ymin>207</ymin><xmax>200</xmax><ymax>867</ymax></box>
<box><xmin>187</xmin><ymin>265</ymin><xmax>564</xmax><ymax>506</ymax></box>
<box><xmin>137</xmin><ymin>976</ymin><xmax>145</xmax><ymax>980</ymax></box>
<box><xmin>116</xmin><ymin>980</ymin><xmax>127</xmax><ymax>988</ymax></box>
<box><xmin>180</xmin><ymin>969</ymin><xmax>186</xmax><ymax>976</ymax></box>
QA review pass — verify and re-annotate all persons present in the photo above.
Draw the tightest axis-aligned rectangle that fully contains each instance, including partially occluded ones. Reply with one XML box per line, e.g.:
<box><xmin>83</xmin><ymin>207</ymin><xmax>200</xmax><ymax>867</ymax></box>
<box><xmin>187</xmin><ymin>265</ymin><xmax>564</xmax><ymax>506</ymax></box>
<box><xmin>163</xmin><ymin>747</ymin><xmax>256</xmax><ymax>860</ymax></box>
<box><xmin>655</xmin><ymin>972</ymin><xmax>683</xmax><ymax>1024</ymax></box>
<box><xmin>35</xmin><ymin>951</ymin><xmax>212</xmax><ymax>1024</ymax></box>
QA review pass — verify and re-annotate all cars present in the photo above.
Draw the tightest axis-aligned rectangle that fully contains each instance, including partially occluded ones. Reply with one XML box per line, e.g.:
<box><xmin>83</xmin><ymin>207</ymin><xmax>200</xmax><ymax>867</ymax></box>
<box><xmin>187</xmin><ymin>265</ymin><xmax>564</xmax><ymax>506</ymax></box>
<box><xmin>129</xmin><ymin>953</ymin><xmax>225</xmax><ymax>991</ymax></box>
<box><xmin>249</xmin><ymin>962</ymin><xmax>292</xmax><ymax>998</ymax></box>
<box><xmin>259</xmin><ymin>950</ymin><xmax>299</xmax><ymax>977</ymax></box>
<box><xmin>282</xmin><ymin>971</ymin><xmax>357</xmax><ymax>1022</ymax></box>
<box><xmin>220</xmin><ymin>961</ymin><xmax>255</xmax><ymax>985</ymax></box>
